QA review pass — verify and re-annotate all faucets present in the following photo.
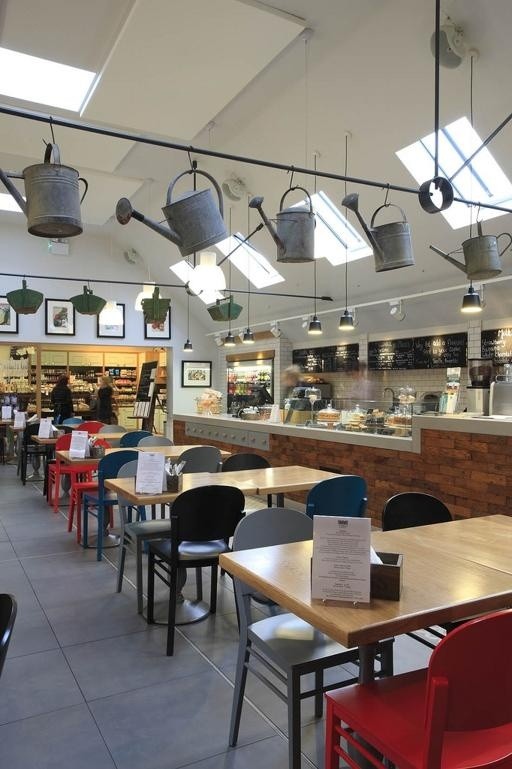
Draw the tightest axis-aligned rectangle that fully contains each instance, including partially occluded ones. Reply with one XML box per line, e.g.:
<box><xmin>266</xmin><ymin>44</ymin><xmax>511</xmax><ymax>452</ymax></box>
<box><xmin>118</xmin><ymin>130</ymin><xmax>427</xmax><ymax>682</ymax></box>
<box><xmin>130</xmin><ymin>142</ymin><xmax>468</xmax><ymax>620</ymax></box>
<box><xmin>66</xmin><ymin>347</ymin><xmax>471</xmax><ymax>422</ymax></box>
<box><xmin>382</xmin><ymin>387</ymin><xmax>394</xmax><ymax>412</ymax></box>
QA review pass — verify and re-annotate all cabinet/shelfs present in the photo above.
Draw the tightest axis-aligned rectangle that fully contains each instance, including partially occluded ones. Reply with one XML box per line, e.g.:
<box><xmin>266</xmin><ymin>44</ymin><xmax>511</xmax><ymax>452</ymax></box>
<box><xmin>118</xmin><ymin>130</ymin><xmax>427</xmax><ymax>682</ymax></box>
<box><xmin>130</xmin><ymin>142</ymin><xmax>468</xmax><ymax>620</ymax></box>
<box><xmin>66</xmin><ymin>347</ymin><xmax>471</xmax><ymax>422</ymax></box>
<box><xmin>102</xmin><ymin>366</ymin><xmax>138</xmax><ymax>395</ymax></box>
<box><xmin>67</xmin><ymin>366</ymin><xmax>104</xmax><ymax>382</ymax></box>
<box><xmin>29</xmin><ymin>365</ymin><xmax>68</xmax><ymax>390</ymax></box>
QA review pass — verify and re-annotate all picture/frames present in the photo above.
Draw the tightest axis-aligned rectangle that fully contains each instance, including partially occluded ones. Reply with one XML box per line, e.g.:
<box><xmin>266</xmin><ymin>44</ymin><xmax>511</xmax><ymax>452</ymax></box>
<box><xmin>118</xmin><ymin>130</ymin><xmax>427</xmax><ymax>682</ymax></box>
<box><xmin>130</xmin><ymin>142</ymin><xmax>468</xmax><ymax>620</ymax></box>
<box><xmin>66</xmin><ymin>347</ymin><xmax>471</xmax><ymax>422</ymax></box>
<box><xmin>181</xmin><ymin>360</ymin><xmax>212</xmax><ymax>388</ymax></box>
<box><xmin>144</xmin><ymin>305</ymin><xmax>171</xmax><ymax>340</ymax></box>
<box><xmin>0</xmin><ymin>296</ymin><xmax>19</xmax><ymax>334</ymax></box>
<box><xmin>44</xmin><ymin>298</ymin><xmax>75</xmax><ymax>336</ymax></box>
<box><xmin>96</xmin><ymin>303</ymin><xmax>125</xmax><ymax>339</ymax></box>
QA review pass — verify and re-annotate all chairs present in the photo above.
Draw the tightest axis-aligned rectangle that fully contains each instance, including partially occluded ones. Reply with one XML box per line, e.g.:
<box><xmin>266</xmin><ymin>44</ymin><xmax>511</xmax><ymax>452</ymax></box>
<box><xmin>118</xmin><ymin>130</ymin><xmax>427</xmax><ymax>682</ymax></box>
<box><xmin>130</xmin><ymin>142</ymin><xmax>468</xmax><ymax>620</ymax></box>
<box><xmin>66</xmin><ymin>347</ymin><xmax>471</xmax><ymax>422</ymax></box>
<box><xmin>67</xmin><ymin>439</ymin><xmax>113</xmax><ymax>544</ymax></box>
<box><xmin>0</xmin><ymin>593</ymin><xmax>17</xmax><ymax>681</ymax></box>
<box><xmin>178</xmin><ymin>446</ymin><xmax>223</xmax><ymax>473</ymax></box>
<box><xmin>19</xmin><ymin>423</ymin><xmax>57</xmax><ymax>486</ymax></box>
<box><xmin>145</xmin><ymin>484</ymin><xmax>245</xmax><ymax>657</ymax></box>
<box><xmin>323</xmin><ymin>610</ymin><xmax>512</xmax><ymax>769</ymax></box>
<box><xmin>227</xmin><ymin>506</ymin><xmax>396</xmax><ymax>769</ymax></box>
<box><xmin>77</xmin><ymin>422</ymin><xmax>106</xmax><ymax>434</ymax></box>
<box><xmin>116</xmin><ymin>459</ymin><xmax>186</xmax><ymax>613</ymax></box>
<box><xmin>381</xmin><ymin>491</ymin><xmax>472</xmax><ymax>650</ymax></box>
<box><xmin>217</xmin><ymin>453</ymin><xmax>284</xmax><ymax>579</ymax></box>
<box><xmin>62</xmin><ymin>418</ymin><xmax>85</xmax><ymax>424</ymax></box>
<box><xmin>98</xmin><ymin>424</ymin><xmax>128</xmax><ymax>433</ymax></box>
<box><xmin>82</xmin><ymin>451</ymin><xmax>146</xmax><ymax>561</ymax></box>
<box><xmin>47</xmin><ymin>433</ymin><xmax>98</xmax><ymax>513</ymax></box>
<box><xmin>305</xmin><ymin>474</ymin><xmax>369</xmax><ymax>521</ymax></box>
<box><xmin>138</xmin><ymin>436</ymin><xmax>174</xmax><ymax>447</ymax></box>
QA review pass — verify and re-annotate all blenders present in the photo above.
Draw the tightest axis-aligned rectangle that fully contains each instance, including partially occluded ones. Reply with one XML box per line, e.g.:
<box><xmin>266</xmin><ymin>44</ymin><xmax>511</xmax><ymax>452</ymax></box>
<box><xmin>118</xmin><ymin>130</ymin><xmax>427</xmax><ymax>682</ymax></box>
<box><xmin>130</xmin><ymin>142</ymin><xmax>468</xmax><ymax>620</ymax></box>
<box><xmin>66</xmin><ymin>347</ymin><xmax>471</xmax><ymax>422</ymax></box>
<box><xmin>465</xmin><ymin>357</ymin><xmax>493</xmax><ymax>416</ymax></box>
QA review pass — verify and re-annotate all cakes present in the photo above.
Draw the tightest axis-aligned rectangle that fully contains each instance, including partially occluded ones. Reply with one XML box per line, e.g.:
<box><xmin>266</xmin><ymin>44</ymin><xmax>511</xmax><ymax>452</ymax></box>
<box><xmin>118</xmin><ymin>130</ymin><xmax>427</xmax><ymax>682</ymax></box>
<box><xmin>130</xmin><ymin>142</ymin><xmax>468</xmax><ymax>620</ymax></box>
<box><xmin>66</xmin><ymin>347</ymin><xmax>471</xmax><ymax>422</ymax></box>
<box><xmin>317</xmin><ymin>411</ymin><xmax>340</xmax><ymax>422</ymax></box>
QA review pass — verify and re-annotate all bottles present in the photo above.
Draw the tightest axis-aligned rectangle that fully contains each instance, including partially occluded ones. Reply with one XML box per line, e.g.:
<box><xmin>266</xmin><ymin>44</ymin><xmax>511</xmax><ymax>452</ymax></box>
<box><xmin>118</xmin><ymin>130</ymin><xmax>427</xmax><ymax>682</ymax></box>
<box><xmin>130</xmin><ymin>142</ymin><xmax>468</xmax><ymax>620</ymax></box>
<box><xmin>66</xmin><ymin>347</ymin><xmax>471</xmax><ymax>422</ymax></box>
<box><xmin>76</xmin><ymin>368</ymin><xmax>95</xmax><ymax>380</ymax></box>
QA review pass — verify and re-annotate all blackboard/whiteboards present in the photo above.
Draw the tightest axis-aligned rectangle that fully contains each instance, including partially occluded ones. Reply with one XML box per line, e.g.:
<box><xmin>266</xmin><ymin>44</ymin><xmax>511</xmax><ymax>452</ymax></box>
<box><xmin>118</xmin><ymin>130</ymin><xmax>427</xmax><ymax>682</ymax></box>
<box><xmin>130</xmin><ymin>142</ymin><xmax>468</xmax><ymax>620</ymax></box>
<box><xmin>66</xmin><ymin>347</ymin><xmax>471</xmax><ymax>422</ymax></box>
<box><xmin>292</xmin><ymin>343</ymin><xmax>359</xmax><ymax>373</ymax></box>
<box><xmin>136</xmin><ymin>361</ymin><xmax>157</xmax><ymax>402</ymax></box>
<box><xmin>367</xmin><ymin>331</ymin><xmax>468</xmax><ymax>370</ymax></box>
<box><xmin>480</xmin><ymin>327</ymin><xmax>511</xmax><ymax>364</ymax></box>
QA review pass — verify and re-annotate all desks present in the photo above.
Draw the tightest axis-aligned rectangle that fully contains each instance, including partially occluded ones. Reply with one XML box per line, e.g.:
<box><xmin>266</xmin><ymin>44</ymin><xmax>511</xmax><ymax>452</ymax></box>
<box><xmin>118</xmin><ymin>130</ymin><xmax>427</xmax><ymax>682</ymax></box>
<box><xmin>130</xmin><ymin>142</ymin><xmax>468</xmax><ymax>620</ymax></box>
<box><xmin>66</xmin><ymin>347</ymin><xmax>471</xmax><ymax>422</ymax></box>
<box><xmin>104</xmin><ymin>465</ymin><xmax>360</xmax><ymax>615</ymax></box>
<box><xmin>220</xmin><ymin>513</ymin><xmax>512</xmax><ymax>769</ymax></box>
<box><xmin>55</xmin><ymin>444</ymin><xmax>239</xmax><ymax>551</ymax></box>
<box><xmin>31</xmin><ymin>433</ymin><xmax>127</xmax><ymax>496</ymax></box>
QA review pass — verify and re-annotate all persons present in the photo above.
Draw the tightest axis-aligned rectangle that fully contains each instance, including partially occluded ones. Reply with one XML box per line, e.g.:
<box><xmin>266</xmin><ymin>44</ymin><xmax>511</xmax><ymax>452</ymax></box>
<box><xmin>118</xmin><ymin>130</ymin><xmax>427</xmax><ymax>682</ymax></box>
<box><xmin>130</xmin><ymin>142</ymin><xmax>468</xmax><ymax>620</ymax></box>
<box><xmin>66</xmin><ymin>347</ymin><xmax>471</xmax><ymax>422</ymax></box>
<box><xmin>98</xmin><ymin>379</ymin><xmax>114</xmax><ymax>424</ymax></box>
<box><xmin>51</xmin><ymin>375</ymin><xmax>75</xmax><ymax>425</ymax></box>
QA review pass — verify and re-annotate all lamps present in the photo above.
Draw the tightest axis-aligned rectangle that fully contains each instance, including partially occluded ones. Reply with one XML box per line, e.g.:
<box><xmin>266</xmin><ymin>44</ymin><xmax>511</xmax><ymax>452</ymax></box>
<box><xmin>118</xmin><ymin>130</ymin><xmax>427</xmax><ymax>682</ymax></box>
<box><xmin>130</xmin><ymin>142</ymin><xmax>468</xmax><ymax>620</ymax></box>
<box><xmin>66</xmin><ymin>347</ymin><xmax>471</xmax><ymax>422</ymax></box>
<box><xmin>184</xmin><ymin>339</ymin><xmax>192</xmax><ymax>353</ymax></box>
<box><xmin>242</xmin><ymin>327</ymin><xmax>255</xmax><ymax>345</ymax></box>
<box><xmin>309</xmin><ymin>314</ymin><xmax>322</xmax><ymax>336</ymax></box>
<box><xmin>339</xmin><ymin>310</ymin><xmax>355</xmax><ymax>333</ymax></box>
<box><xmin>458</xmin><ymin>288</ymin><xmax>482</xmax><ymax>319</ymax></box>
<box><xmin>224</xmin><ymin>332</ymin><xmax>235</xmax><ymax>349</ymax></box>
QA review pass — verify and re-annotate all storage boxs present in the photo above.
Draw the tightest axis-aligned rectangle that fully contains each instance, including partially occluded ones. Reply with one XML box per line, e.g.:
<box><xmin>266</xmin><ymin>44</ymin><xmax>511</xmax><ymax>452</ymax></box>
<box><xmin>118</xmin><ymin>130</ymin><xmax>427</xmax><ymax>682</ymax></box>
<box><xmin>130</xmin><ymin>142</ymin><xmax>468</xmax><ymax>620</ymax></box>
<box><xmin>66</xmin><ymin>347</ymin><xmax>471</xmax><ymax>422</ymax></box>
<box><xmin>119</xmin><ymin>431</ymin><xmax>155</xmax><ymax>448</ymax></box>
